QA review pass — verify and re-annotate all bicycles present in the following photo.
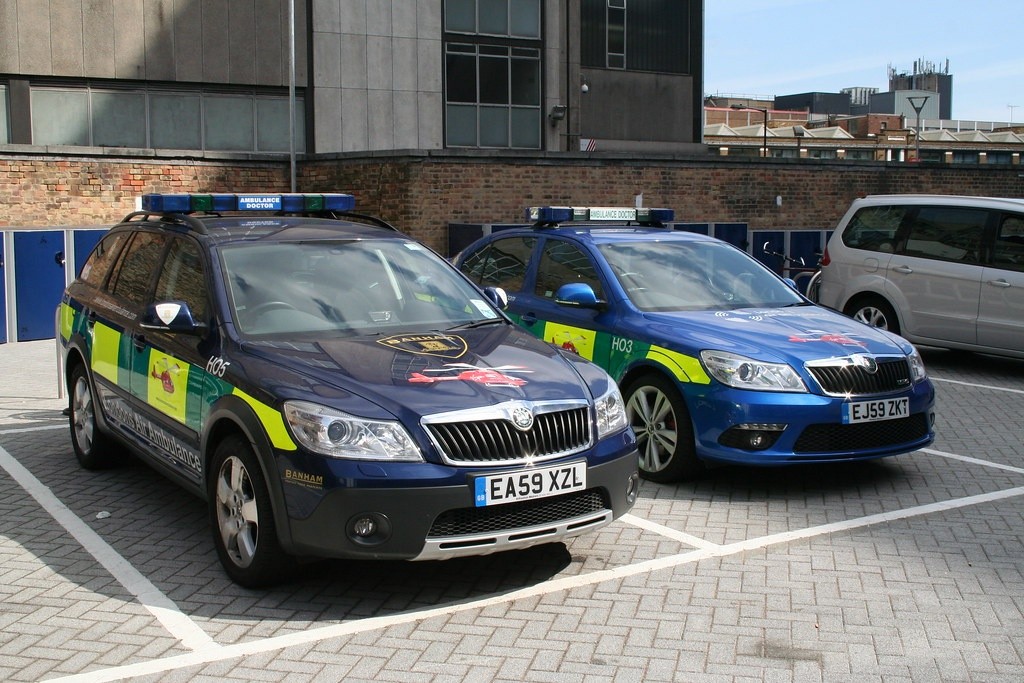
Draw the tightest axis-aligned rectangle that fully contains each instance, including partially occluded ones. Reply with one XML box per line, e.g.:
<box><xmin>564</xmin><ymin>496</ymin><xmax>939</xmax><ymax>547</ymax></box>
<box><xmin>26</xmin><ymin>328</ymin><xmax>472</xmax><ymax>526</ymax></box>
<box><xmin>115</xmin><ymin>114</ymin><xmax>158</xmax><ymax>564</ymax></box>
<box><xmin>763</xmin><ymin>241</ymin><xmax>823</xmax><ymax>307</ymax></box>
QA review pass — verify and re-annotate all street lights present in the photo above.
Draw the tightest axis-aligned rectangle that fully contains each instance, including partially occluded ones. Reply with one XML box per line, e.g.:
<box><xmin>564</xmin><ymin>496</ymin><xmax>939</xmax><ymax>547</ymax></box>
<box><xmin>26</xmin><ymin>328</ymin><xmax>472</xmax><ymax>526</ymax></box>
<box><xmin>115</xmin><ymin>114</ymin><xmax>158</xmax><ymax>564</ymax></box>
<box><xmin>730</xmin><ymin>103</ymin><xmax>767</xmax><ymax>158</ymax></box>
<box><xmin>907</xmin><ymin>95</ymin><xmax>930</xmax><ymax>163</ymax></box>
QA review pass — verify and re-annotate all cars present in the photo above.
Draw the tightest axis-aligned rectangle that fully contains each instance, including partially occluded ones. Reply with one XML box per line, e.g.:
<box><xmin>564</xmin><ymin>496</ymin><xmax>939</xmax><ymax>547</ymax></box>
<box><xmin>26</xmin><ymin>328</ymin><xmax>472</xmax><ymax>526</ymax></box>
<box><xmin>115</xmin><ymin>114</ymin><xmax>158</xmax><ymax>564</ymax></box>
<box><xmin>410</xmin><ymin>205</ymin><xmax>936</xmax><ymax>485</ymax></box>
<box><xmin>818</xmin><ymin>194</ymin><xmax>1024</xmax><ymax>364</ymax></box>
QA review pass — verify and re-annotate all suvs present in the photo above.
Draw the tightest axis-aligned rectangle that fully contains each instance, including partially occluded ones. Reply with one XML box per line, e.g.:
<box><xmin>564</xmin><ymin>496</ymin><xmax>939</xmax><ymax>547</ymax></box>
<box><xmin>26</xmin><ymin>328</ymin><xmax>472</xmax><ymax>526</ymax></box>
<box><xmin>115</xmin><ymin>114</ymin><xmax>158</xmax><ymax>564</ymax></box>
<box><xmin>56</xmin><ymin>192</ymin><xmax>643</xmax><ymax>590</ymax></box>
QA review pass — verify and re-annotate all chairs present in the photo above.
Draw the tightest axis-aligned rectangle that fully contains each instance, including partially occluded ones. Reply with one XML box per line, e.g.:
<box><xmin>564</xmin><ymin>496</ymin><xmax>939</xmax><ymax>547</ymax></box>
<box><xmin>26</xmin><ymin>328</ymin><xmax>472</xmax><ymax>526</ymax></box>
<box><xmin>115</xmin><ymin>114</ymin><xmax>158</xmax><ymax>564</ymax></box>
<box><xmin>187</xmin><ymin>259</ymin><xmax>381</xmax><ymax>327</ymax></box>
<box><xmin>471</xmin><ymin>251</ymin><xmax>716</xmax><ymax>309</ymax></box>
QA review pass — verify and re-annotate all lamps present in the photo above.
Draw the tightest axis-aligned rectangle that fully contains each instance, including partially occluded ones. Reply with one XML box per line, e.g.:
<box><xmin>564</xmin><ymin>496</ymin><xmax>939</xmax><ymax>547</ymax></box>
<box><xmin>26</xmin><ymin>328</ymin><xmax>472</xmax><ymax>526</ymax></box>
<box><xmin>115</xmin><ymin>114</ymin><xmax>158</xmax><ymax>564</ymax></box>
<box><xmin>547</xmin><ymin>104</ymin><xmax>568</xmax><ymax>127</ymax></box>
<box><xmin>792</xmin><ymin>126</ymin><xmax>804</xmax><ymax>156</ymax></box>
